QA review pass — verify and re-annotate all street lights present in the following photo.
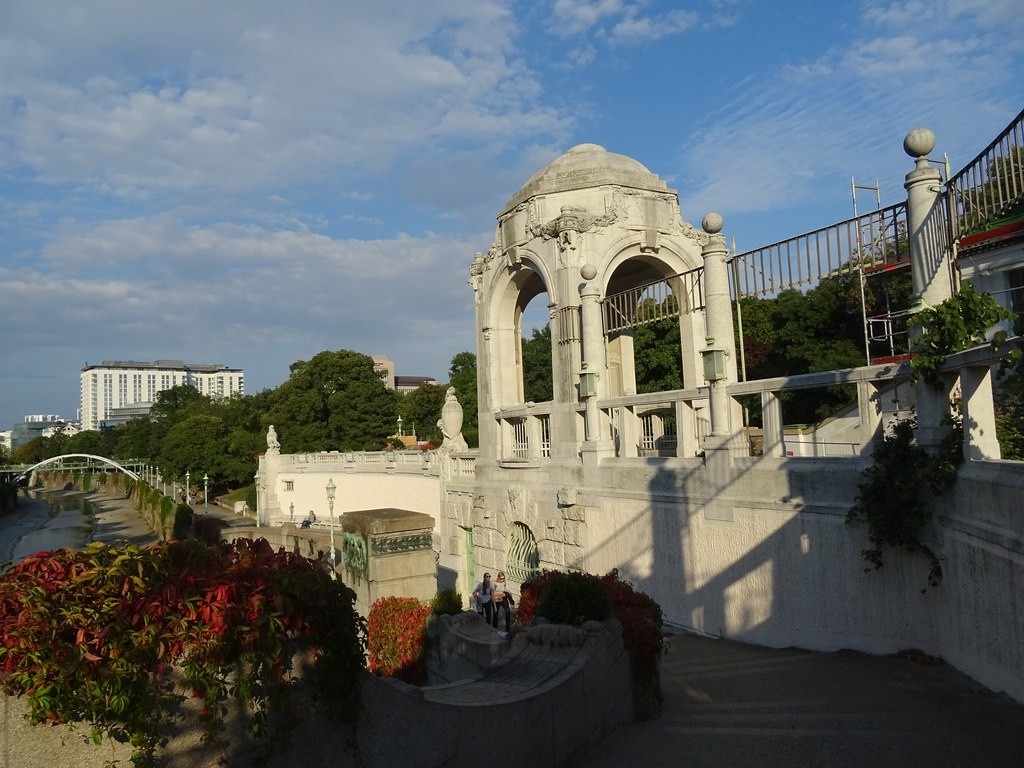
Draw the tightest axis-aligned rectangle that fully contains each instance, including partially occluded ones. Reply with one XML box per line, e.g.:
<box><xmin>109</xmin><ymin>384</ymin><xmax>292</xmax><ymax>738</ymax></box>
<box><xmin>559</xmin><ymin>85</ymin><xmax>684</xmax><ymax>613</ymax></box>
<box><xmin>150</xmin><ymin>465</ymin><xmax>154</xmax><ymax>487</ymax></box>
<box><xmin>325</xmin><ymin>476</ymin><xmax>338</xmax><ymax>570</ymax></box>
<box><xmin>141</xmin><ymin>464</ymin><xmax>144</xmax><ymax>482</ymax></box>
<box><xmin>155</xmin><ymin>466</ymin><xmax>160</xmax><ymax>489</ymax></box>
<box><xmin>146</xmin><ymin>464</ymin><xmax>149</xmax><ymax>483</ymax></box>
<box><xmin>202</xmin><ymin>473</ymin><xmax>209</xmax><ymax>514</ymax></box>
<box><xmin>396</xmin><ymin>414</ymin><xmax>403</xmax><ymax>437</ymax></box>
<box><xmin>184</xmin><ymin>471</ymin><xmax>190</xmax><ymax>505</ymax></box>
<box><xmin>253</xmin><ymin>471</ymin><xmax>262</xmax><ymax>527</ymax></box>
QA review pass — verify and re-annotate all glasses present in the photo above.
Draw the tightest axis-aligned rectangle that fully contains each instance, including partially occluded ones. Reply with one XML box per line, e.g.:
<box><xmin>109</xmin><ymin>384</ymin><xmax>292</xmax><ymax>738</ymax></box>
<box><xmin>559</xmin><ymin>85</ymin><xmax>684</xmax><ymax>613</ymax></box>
<box><xmin>486</xmin><ymin>576</ymin><xmax>491</xmax><ymax>578</ymax></box>
<box><xmin>499</xmin><ymin>578</ymin><xmax>505</xmax><ymax>580</ymax></box>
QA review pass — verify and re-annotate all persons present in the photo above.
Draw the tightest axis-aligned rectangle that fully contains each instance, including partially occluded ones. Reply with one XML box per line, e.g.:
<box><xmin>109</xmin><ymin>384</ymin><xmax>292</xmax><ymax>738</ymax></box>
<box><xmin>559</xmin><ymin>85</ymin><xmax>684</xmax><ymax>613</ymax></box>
<box><xmin>178</xmin><ymin>487</ymin><xmax>195</xmax><ymax>505</ymax></box>
<box><xmin>491</xmin><ymin>572</ymin><xmax>510</xmax><ymax>632</ymax></box>
<box><xmin>302</xmin><ymin>511</ymin><xmax>315</xmax><ymax>528</ymax></box>
<box><xmin>473</xmin><ymin>573</ymin><xmax>493</xmax><ymax>624</ymax></box>
